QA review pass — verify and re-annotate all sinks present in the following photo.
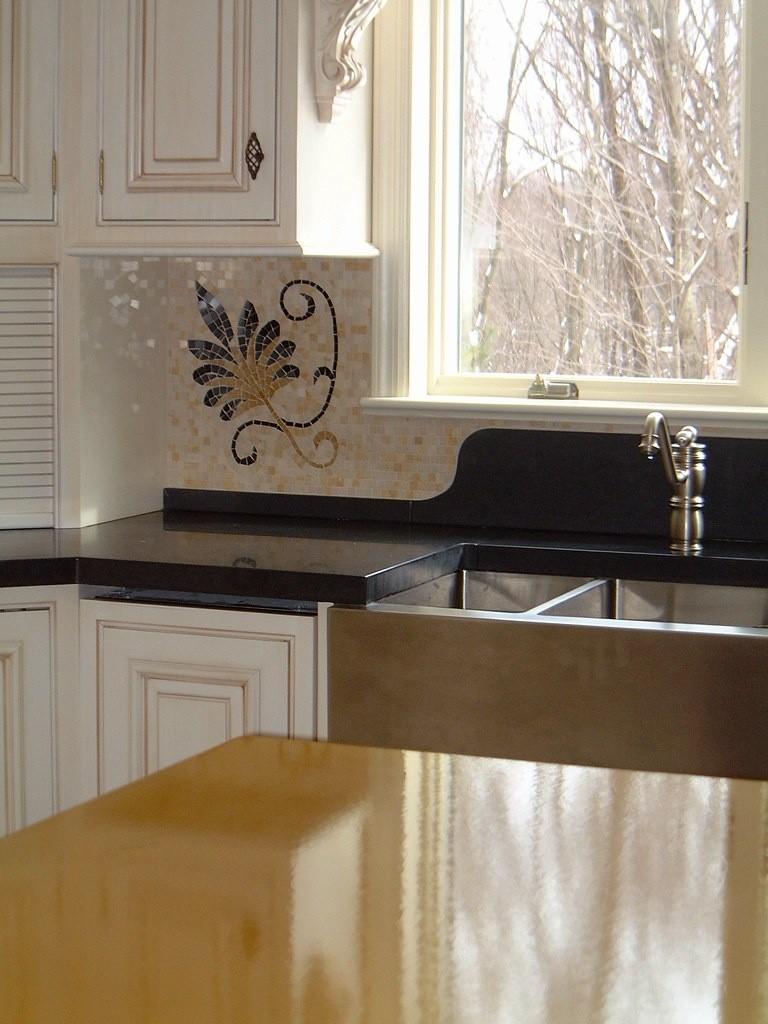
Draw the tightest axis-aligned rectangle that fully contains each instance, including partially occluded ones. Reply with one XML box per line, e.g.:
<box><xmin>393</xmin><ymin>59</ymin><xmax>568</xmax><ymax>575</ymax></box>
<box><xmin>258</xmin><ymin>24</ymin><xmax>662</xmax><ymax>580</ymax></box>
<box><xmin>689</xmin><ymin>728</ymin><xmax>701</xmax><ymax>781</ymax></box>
<box><xmin>536</xmin><ymin>574</ymin><xmax>768</xmax><ymax>636</ymax></box>
<box><xmin>366</xmin><ymin>564</ymin><xmax>599</xmax><ymax>625</ymax></box>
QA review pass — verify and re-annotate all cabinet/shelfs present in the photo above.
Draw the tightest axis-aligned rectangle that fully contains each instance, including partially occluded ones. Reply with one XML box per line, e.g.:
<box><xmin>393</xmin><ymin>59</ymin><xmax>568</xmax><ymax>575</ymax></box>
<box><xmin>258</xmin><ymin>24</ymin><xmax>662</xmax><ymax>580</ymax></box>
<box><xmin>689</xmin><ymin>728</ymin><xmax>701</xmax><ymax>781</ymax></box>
<box><xmin>56</xmin><ymin>0</ymin><xmax>380</xmax><ymax>259</ymax></box>
<box><xmin>1</xmin><ymin>1</ymin><xmax>56</xmax><ymax>265</ymax></box>
<box><xmin>0</xmin><ymin>583</ymin><xmax>83</xmax><ymax>840</ymax></box>
<box><xmin>78</xmin><ymin>598</ymin><xmax>319</xmax><ymax>808</ymax></box>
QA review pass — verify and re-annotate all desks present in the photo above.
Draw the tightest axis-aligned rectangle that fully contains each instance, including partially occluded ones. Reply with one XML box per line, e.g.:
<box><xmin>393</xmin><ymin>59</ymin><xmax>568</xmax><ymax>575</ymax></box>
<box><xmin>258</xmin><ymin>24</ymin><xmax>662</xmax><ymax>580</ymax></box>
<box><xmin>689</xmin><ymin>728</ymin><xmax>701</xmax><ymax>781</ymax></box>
<box><xmin>0</xmin><ymin>733</ymin><xmax>768</xmax><ymax>1023</ymax></box>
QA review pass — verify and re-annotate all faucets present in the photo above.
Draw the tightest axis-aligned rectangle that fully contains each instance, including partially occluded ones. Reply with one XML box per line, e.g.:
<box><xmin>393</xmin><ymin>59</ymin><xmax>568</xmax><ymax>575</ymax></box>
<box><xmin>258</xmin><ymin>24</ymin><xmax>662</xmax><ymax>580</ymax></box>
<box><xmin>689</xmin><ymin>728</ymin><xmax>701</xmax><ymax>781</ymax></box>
<box><xmin>636</xmin><ymin>409</ymin><xmax>710</xmax><ymax>553</ymax></box>
